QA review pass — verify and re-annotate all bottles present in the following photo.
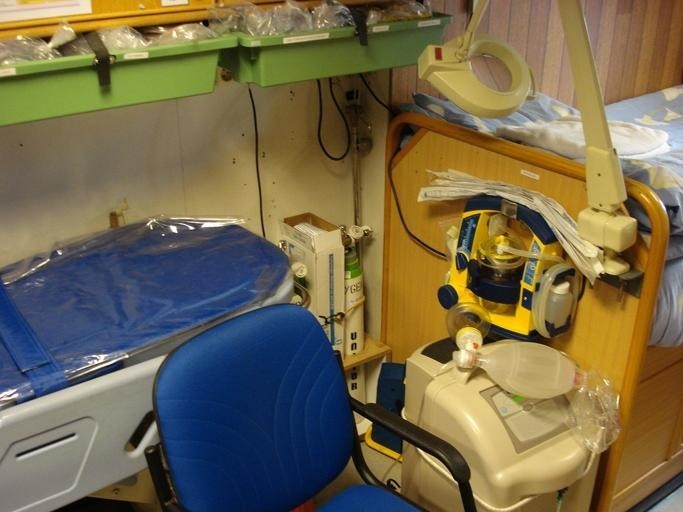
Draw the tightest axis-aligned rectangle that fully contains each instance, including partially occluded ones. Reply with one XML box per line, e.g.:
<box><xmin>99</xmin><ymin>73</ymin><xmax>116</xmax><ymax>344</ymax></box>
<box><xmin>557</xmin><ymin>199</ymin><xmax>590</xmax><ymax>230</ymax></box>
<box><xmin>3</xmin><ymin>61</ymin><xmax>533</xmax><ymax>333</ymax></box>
<box><xmin>545</xmin><ymin>279</ymin><xmax>573</xmax><ymax>331</ymax></box>
<box><xmin>452</xmin><ymin>339</ymin><xmax>576</xmax><ymax>401</ymax></box>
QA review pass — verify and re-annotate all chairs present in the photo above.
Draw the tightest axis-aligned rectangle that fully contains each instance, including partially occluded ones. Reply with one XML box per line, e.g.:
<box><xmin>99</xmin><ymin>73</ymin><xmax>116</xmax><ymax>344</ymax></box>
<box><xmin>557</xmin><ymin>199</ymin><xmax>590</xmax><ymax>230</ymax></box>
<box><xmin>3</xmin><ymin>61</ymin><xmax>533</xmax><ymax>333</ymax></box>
<box><xmin>143</xmin><ymin>302</ymin><xmax>480</xmax><ymax>512</ymax></box>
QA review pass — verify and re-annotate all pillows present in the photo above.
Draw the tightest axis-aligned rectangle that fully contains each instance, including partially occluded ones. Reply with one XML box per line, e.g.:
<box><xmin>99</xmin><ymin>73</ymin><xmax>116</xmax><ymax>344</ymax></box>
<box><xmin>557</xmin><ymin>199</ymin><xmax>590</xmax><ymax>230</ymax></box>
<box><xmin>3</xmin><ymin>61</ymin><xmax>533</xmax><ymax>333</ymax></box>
<box><xmin>401</xmin><ymin>89</ymin><xmax>683</xmax><ymax>512</ymax></box>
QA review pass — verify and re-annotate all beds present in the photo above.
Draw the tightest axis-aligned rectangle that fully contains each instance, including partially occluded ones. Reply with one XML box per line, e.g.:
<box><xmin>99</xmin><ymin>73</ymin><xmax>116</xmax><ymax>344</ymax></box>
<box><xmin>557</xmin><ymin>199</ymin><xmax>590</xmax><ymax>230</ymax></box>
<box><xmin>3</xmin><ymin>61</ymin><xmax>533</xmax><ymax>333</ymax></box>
<box><xmin>383</xmin><ymin>83</ymin><xmax>683</xmax><ymax>512</ymax></box>
<box><xmin>0</xmin><ymin>216</ymin><xmax>313</xmax><ymax>512</ymax></box>
